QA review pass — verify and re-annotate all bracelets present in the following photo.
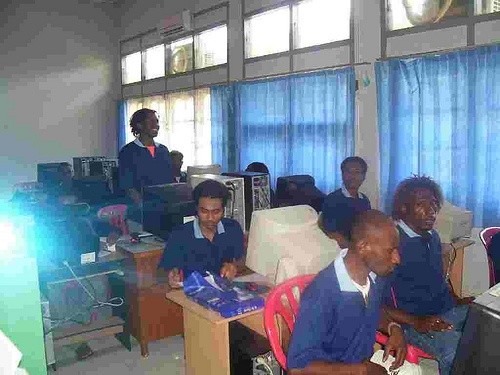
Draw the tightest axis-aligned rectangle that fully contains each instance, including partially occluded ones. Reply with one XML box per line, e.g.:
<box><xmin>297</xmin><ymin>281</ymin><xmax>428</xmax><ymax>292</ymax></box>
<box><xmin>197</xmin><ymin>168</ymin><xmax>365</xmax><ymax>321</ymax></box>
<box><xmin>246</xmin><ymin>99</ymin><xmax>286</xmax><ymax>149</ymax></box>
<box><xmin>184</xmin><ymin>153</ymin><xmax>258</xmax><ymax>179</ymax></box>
<box><xmin>387</xmin><ymin>322</ymin><xmax>401</xmax><ymax>336</ymax></box>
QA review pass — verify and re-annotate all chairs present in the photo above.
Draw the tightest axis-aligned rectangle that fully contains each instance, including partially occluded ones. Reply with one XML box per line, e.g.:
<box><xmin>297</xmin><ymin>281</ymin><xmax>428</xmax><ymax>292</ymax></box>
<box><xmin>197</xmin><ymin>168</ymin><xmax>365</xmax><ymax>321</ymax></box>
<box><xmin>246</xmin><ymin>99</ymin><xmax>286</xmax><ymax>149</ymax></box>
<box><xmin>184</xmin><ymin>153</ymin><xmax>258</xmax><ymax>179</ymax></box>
<box><xmin>479</xmin><ymin>226</ymin><xmax>500</xmax><ymax>290</ymax></box>
<box><xmin>265</xmin><ymin>274</ymin><xmax>435</xmax><ymax>375</ymax></box>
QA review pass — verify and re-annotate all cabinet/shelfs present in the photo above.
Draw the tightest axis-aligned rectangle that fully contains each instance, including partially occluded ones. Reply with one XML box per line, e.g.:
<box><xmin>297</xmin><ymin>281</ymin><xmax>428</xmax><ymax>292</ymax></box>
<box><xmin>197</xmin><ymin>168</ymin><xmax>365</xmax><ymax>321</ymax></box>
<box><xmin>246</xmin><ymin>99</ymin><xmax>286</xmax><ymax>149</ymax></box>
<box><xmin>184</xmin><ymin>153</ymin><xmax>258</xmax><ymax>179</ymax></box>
<box><xmin>37</xmin><ymin>248</ymin><xmax>131</xmax><ymax>370</ymax></box>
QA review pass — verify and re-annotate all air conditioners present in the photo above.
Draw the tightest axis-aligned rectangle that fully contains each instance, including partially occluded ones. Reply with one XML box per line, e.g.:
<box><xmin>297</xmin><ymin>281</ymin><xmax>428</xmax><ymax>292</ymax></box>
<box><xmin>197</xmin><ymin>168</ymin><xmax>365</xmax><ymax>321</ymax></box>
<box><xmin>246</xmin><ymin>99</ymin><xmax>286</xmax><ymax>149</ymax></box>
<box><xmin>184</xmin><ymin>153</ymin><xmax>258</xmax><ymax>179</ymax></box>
<box><xmin>420</xmin><ymin>0</ymin><xmax>500</xmax><ymax>23</ymax></box>
<box><xmin>173</xmin><ymin>42</ymin><xmax>203</xmax><ymax>73</ymax></box>
<box><xmin>154</xmin><ymin>10</ymin><xmax>193</xmax><ymax>39</ymax></box>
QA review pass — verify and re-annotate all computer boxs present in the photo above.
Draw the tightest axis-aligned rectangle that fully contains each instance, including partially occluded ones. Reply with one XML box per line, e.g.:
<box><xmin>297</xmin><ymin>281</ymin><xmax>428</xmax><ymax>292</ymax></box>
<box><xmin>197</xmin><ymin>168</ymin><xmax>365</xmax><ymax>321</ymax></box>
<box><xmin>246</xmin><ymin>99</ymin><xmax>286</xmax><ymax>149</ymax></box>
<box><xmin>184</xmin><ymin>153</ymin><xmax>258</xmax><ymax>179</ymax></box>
<box><xmin>189</xmin><ymin>173</ymin><xmax>245</xmax><ymax>234</ymax></box>
<box><xmin>73</xmin><ymin>156</ymin><xmax>120</xmax><ymax>198</ymax></box>
<box><xmin>222</xmin><ymin>171</ymin><xmax>271</xmax><ymax>231</ymax></box>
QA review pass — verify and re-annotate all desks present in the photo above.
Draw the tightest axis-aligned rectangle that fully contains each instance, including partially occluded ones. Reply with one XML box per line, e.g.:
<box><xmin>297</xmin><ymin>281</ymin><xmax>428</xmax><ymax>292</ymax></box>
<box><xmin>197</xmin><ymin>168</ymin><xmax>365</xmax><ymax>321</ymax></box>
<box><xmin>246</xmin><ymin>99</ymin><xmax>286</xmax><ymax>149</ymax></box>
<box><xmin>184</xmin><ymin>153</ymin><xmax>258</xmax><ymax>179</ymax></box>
<box><xmin>167</xmin><ymin>271</ymin><xmax>275</xmax><ymax>375</ymax></box>
<box><xmin>438</xmin><ymin>237</ymin><xmax>476</xmax><ymax>298</ymax></box>
<box><xmin>112</xmin><ymin>237</ymin><xmax>185</xmax><ymax>357</ymax></box>
<box><xmin>472</xmin><ymin>282</ymin><xmax>499</xmax><ymax>374</ymax></box>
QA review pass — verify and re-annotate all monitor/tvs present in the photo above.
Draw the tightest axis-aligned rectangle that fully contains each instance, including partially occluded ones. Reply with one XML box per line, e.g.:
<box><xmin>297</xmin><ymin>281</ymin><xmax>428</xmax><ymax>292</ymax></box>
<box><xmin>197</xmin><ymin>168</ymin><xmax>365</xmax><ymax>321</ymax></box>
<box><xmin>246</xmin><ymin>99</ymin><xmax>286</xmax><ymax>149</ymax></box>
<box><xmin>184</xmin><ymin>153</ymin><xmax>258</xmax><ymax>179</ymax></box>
<box><xmin>37</xmin><ymin>162</ymin><xmax>73</xmax><ymax>193</ymax></box>
<box><xmin>33</xmin><ymin>203</ymin><xmax>100</xmax><ymax>267</ymax></box>
<box><xmin>431</xmin><ymin>200</ymin><xmax>472</xmax><ymax>244</ymax></box>
<box><xmin>449</xmin><ymin>282</ymin><xmax>500</xmax><ymax>375</ymax></box>
<box><xmin>187</xmin><ymin>165</ymin><xmax>221</xmax><ymax>185</ymax></box>
<box><xmin>245</xmin><ymin>204</ymin><xmax>341</xmax><ymax>286</ymax></box>
<box><xmin>273</xmin><ymin>175</ymin><xmax>328</xmax><ymax>214</ymax></box>
<box><xmin>72</xmin><ymin>176</ymin><xmax>110</xmax><ymax>207</ymax></box>
<box><xmin>142</xmin><ymin>182</ymin><xmax>192</xmax><ymax>240</ymax></box>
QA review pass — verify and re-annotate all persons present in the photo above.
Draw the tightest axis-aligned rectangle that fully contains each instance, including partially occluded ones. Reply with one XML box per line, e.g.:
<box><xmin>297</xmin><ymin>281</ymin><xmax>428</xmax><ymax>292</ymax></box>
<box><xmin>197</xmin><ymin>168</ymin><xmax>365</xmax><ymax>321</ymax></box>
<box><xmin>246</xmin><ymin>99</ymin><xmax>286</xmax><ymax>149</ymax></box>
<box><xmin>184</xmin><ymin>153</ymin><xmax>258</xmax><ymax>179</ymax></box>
<box><xmin>170</xmin><ymin>149</ymin><xmax>188</xmax><ymax>182</ymax></box>
<box><xmin>323</xmin><ymin>157</ymin><xmax>371</xmax><ymax>247</ymax></box>
<box><xmin>244</xmin><ymin>162</ymin><xmax>275</xmax><ymax>211</ymax></box>
<box><xmin>382</xmin><ymin>173</ymin><xmax>476</xmax><ymax>375</ymax></box>
<box><xmin>286</xmin><ymin>209</ymin><xmax>409</xmax><ymax>375</ymax></box>
<box><xmin>156</xmin><ymin>180</ymin><xmax>245</xmax><ymax>291</ymax></box>
<box><xmin>51</xmin><ymin>162</ymin><xmax>82</xmax><ymax>203</ymax></box>
<box><xmin>118</xmin><ymin>109</ymin><xmax>177</xmax><ymax>232</ymax></box>
